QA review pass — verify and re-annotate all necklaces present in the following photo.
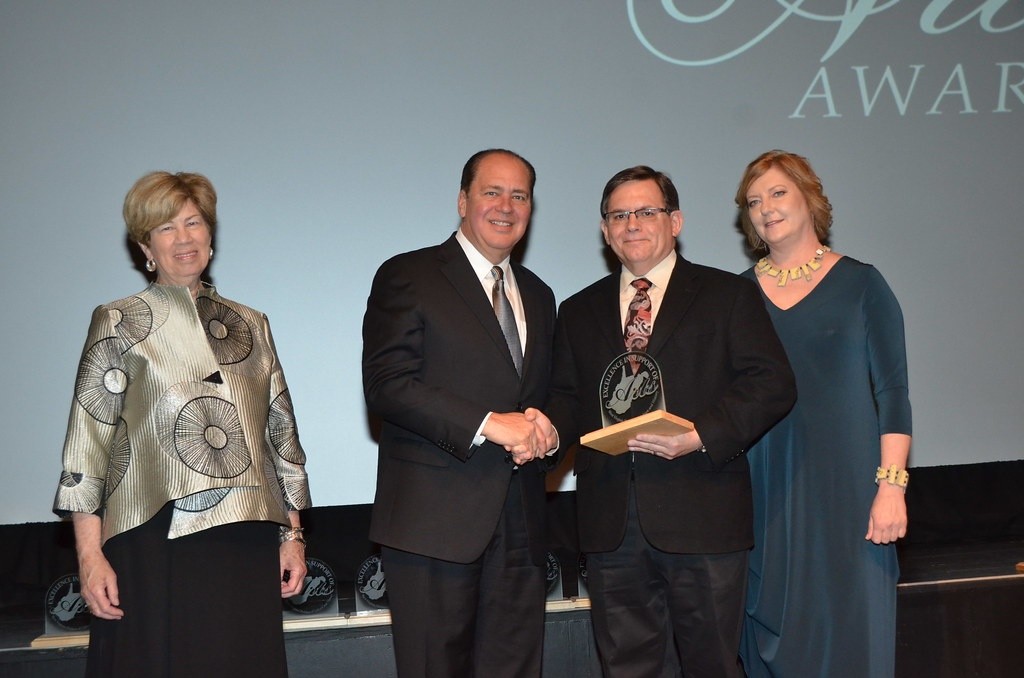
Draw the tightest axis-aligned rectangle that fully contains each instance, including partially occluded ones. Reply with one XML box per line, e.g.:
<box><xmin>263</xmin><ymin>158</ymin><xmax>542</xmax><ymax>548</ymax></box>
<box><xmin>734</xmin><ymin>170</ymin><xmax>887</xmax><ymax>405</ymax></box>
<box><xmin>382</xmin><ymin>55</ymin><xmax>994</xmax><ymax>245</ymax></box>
<box><xmin>755</xmin><ymin>245</ymin><xmax>831</xmax><ymax>286</ymax></box>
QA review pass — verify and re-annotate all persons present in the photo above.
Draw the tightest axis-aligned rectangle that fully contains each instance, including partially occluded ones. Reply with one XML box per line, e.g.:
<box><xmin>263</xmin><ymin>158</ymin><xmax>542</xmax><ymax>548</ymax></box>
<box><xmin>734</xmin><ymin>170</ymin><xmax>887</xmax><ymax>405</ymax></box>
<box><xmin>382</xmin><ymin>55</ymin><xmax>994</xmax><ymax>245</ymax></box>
<box><xmin>503</xmin><ymin>165</ymin><xmax>800</xmax><ymax>678</ymax></box>
<box><xmin>360</xmin><ymin>148</ymin><xmax>559</xmax><ymax>678</ymax></box>
<box><xmin>52</xmin><ymin>170</ymin><xmax>314</xmax><ymax>678</ymax></box>
<box><xmin>734</xmin><ymin>148</ymin><xmax>912</xmax><ymax>678</ymax></box>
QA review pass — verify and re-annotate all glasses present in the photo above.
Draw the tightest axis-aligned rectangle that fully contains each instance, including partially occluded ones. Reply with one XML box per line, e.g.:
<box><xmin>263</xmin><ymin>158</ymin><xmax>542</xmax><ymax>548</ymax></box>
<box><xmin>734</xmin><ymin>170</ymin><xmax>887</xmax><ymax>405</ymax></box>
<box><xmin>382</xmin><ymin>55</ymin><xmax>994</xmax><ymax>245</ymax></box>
<box><xmin>602</xmin><ymin>208</ymin><xmax>667</xmax><ymax>225</ymax></box>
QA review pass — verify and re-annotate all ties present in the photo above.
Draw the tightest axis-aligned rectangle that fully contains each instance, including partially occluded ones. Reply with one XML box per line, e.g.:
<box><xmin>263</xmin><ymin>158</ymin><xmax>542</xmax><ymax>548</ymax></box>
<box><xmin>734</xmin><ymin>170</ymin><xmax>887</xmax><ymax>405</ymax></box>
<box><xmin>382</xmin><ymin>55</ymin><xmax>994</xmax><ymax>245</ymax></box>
<box><xmin>622</xmin><ymin>278</ymin><xmax>653</xmax><ymax>376</ymax></box>
<box><xmin>490</xmin><ymin>264</ymin><xmax>523</xmax><ymax>378</ymax></box>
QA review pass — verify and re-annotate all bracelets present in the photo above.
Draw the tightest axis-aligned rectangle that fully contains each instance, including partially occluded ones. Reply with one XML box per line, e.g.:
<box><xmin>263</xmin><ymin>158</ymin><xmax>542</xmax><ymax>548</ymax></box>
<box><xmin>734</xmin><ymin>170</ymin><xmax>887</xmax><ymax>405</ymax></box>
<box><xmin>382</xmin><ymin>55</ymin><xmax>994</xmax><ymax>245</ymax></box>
<box><xmin>875</xmin><ymin>464</ymin><xmax>909</xmax><ymax>494</ymax></box>
<box><xmin>278</xmin><ymin>525</ymin><xmax>307</xmax><ymax>547</ymax></box>
